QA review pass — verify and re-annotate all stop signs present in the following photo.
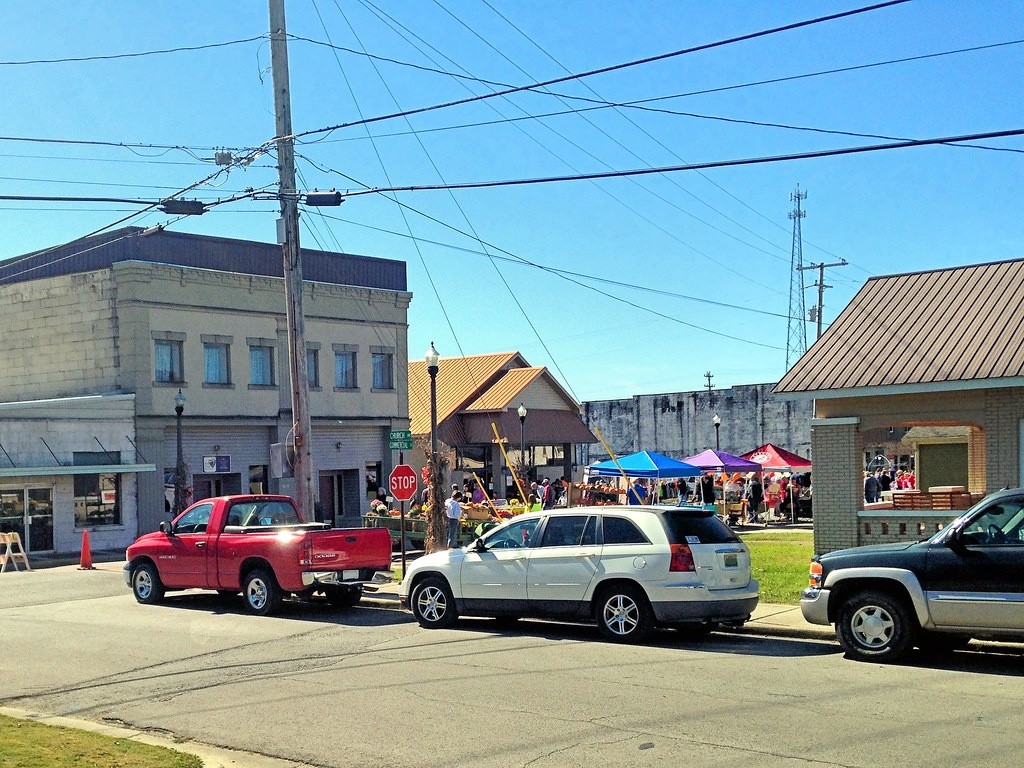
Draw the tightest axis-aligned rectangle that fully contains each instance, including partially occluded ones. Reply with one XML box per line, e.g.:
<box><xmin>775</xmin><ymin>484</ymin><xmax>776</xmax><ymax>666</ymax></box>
<box><xmin>388</xmin><ymin>464</ymin><xmax>418</xmax><ymax>503</ymax></box>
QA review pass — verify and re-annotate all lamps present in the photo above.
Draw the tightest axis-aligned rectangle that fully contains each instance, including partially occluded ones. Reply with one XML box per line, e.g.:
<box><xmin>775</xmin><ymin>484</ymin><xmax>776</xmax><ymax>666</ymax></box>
<box><xmin>337</xmin><ymin>442</ymin><xmax>344</xmax><ymax>452</ymax></box>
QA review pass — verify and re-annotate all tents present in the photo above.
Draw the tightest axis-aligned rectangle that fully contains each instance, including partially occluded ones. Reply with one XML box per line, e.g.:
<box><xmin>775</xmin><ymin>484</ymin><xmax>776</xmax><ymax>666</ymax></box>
<box><xmin>652</xmin><ymin>448</ymin><xmax>766</xmax><ymax>524</ymax></box>
<box><xmin>588</xmin><ymin>450</ymin><xmax>704</xmax><ymax>510</ymax></box>
<box><xmin>739</xmin><ymin>442</ymin><xmax>813</xmax><ymax>523</ymax></box>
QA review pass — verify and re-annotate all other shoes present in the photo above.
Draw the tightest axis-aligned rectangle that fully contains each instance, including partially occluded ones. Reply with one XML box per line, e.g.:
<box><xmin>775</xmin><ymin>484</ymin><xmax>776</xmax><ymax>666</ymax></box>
<box><xmin>748</xmin><ymin>519</ymin><xmax>754</xmax><ymax>523</ymax></box>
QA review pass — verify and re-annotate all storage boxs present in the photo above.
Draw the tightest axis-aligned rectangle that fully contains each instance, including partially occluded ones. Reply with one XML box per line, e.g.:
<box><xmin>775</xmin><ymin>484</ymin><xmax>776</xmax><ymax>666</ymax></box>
<box><xmin>928</xmin><ymin>485</ymin><xmax>965</xmax><ymax>510</ymax></box>
<box><xmin>461</xmin><ymin>506</ymin><xmax>489</xmax><ymax>519</ymax></box>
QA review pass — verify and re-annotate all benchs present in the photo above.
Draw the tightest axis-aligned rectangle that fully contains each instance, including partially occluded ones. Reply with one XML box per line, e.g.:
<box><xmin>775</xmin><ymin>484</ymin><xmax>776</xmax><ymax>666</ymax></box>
<box><xmin>362</xmin><ymin>499</ymin><xmax>494</xmax><ymax>542</ymax></box>
<box><xmin>893</xmin><ymin>493</ymin><xmax>959</xmax><ymax>509</ymax></box>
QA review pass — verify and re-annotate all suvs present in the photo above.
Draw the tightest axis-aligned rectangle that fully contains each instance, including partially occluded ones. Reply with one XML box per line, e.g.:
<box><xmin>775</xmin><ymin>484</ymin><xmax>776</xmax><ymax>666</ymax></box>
<box><xmin>402</xmin><ymin>505</ymin><xmax>763</xmax><ymax>643</ymax></box>
<box><xmin>798</xmin><ymin>486</ymin><xmax>1024</xmax><ymax>663</ymax></box>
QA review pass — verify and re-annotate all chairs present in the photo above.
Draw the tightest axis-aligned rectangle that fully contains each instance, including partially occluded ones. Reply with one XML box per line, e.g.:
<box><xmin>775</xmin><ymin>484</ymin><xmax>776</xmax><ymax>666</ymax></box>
<box><xmin>230</xmin><ymin>513</ymin><xmax>287</xmax><ymax>526</ymax></box>
<box><xmin>497</xmin><ymin>497</ymin><xmax>541</xmax><ymax>515</ymax></box>
<box><xmin>748</xmin><ymin>499</ymin><xmax>813</xmax><ymax>523</ymax></box>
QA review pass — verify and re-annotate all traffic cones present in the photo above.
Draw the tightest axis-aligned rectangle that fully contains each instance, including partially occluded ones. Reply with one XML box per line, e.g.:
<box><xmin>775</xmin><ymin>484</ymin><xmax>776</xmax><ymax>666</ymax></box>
<box><xmin>76</xmin><ymin>529</ymin><xmax>98</xmax><ymax>571</ymax></box>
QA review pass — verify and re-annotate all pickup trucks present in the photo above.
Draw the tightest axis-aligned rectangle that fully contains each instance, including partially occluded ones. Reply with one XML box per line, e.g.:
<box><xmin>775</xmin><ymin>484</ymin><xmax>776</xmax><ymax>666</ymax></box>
<box><xmin>121</xmin><ymin>493</ymin><xmax>394</xmax><ymax>617</ymax></box>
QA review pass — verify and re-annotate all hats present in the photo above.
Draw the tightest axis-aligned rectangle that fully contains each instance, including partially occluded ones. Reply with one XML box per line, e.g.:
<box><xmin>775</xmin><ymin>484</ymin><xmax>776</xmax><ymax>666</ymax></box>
<box><xmin>678</xmin><ymin>478</ymin><xmax>683</xmax><ymax>482</ymax></box>
<box><xmin>541</xmin><ymin>478</ymin><xmax>548</xmax><ymax>484</ymax></box>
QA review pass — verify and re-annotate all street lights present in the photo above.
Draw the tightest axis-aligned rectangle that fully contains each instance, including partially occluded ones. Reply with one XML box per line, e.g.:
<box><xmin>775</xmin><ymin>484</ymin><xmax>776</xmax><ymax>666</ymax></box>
<box><xmin>712</xmin><ymin>413</ymin><xmax>721</xmax><ymax>456</ymax></box>
<box><xmin>517</xmin><ymin>402</ymin><xmax>528</xmax><ymax>496</ymax></box>
<box><xmin>424</xmin><ymin>340</ymin><xmax>446</xmax><ymax>553</ymax></box>
<box><xmin>173</xmin><ymin>389</ymin><xmax>189</xmax><ymax>519</ymax></box>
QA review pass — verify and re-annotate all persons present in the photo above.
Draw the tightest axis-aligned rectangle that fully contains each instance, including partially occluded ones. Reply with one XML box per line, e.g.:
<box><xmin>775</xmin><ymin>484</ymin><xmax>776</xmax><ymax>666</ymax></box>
<box><xmin>374</xmin><ymin>478</ymin><xmax>486</xmax><ymax>548</ymax></box>
<box><xmin>512</xmin><ymin>476</ymin><xmax>569</xmax><ymax>545</ymax></box>
<box><xmin>628</xmin><ymin>474</ymin><xmax>812</xmax><ymax>524</ymax></box>
<box><xmin>863</xmin><ymin>466</ymin><xmax>915</xmax><ymax>506</ymax></box>
